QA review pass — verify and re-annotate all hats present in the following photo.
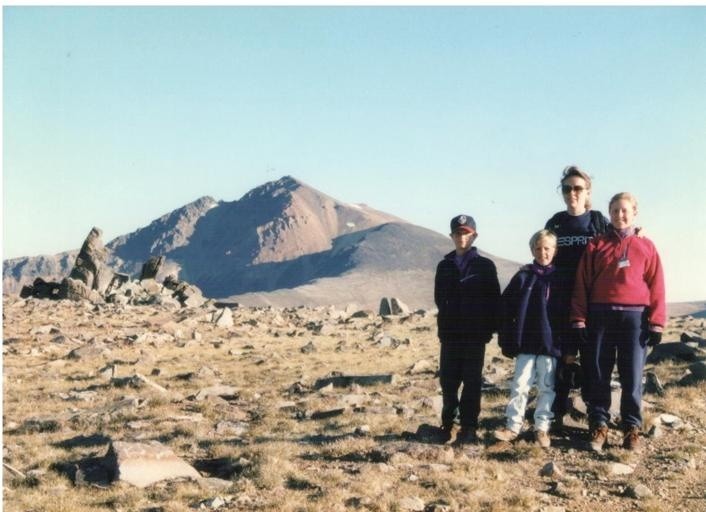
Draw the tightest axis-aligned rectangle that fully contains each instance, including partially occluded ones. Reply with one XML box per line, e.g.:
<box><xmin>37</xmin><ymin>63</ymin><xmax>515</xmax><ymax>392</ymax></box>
<box><xmin>449</xmin><ymin>213</ymin><xmax>477</xmax><ymax>235</ymax></box>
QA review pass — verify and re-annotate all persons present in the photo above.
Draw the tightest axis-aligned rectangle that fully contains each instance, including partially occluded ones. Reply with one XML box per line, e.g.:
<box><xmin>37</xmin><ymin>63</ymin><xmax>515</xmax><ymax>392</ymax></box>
<box><xmin>491</xmin><ymin>226</ymin><xmax>579</xmax><ymax>447</ymax></box>
<box><xmin>427</xmin><ymin>212</ymin><xmax>503</xmax><ymax>447</ymax></box>
<box><xmin>544</xmin><ymin>164</ymin><xmax>611</xmax><ymax>427</ymax></box>
<box><xmin>570</xmin><ymin>191</ymin><xmax>668</xmax><ymax>456</ymax></box>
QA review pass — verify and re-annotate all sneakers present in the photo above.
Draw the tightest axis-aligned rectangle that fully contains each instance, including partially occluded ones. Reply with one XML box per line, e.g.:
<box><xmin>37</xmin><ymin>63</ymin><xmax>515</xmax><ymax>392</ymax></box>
<box><xmin>492</xmin><ymin>427</ymin><xmax>521</xmax><ymax>442</ymax></box>
<box><xmin>622</xmin><ymin>424</ymin><xmax>642</xmax><ymax>451</ymax></box>
<box><xmin>438</xmin><ymin>422</ymin><xmax>477</xmax><ymax>445</ymax></box>
<box><xmin>584</xmin><ymin>425</ymin><xmax>610</xmax><ymax>453</ymax></box>
<box><xmin>533</xmin><ymin>430</ymin><xmax>551</xmax><ymax>448</ymax></box>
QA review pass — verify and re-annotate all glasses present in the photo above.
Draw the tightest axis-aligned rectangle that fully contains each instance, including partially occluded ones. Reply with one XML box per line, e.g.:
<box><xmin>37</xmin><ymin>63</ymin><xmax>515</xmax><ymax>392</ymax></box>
<box><xmin>561</xmin><ymin>183</ymin><xmax>587</xmax><ymax>194</ymax></box>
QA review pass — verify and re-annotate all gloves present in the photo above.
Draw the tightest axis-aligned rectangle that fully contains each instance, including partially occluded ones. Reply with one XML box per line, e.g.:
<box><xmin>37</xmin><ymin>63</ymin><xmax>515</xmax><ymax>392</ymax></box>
<box><xmin>571</xmin><ymin>322</ymin><xmax>590</xmax><ymax>345</ymax></box>
<box><xmin>645</xmin><ymin>326</ymin><xmax>663</xmax><ymax>347</ymax></box>
